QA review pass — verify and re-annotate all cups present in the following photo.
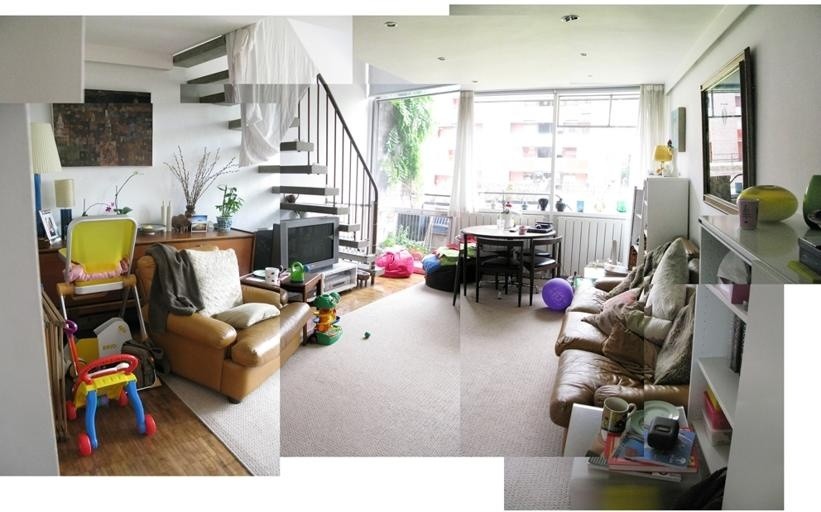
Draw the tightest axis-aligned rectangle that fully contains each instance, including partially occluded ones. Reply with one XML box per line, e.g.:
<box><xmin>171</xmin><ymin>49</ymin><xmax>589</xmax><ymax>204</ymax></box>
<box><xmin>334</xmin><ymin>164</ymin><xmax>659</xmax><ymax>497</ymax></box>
<box><xmin>265</xmin><ymin>267</ymin><xmax>279</xmax><ymax>288</ymax></box>
<box><xmin>600</xmin><ymin>397</ymin><xmax>637</xmax><ymax>435</ymax></box>
<box><xmin>577</xmin><ymin>200</ymin><xmax>584</xmax><ymax>212</ymax></box>
<box><xmin>617</xmin><ymin>199</ymin><xmax>625</xmax><ymax>212</ymax></box>
<box><xmin>538</xmin><ymin>198</ymin><xmax>548</xmax><ymax>211</ymax></box>
<box><xmin>497</xmin><ymin>219</ymin><xmax>505</xmax><ymax>233</ymax></box>
<box><xmin>738</xmin><ymin>198</ymin><xmax>759</xmax><ymax>230</ymax></box>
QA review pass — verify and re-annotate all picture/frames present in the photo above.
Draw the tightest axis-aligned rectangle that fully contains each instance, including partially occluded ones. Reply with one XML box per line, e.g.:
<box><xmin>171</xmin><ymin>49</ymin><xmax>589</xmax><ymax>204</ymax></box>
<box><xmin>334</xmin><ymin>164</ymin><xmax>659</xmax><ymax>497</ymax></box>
<box><xmin>697</xmin><ymin>47</ymin><xmax>755</xmax><ymax>216</ymax></box>
<box><xmin>191</xmin><ymin>214</ymin><xmax>209</xmax><ymax>232</ymax></box>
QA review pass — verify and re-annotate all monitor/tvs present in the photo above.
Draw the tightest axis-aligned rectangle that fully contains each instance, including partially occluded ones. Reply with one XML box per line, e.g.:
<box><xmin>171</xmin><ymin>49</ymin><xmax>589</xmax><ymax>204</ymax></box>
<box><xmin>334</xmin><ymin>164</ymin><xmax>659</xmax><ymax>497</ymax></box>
<box><xmin>270</xmin><ymin>216</ymin><xmax>339</xmax><ymax>272</ymax></box>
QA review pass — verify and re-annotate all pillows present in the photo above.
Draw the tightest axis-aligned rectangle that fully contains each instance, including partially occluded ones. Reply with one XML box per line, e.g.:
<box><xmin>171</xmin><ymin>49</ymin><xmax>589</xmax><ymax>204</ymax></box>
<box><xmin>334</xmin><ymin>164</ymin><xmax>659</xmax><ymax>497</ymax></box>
<box><xmin>211</xmin><ymin>303</ymin><xmax>281</xmax><ymax>330</ymax></box>
<box><xmin>585</xmin><ymin>238</ymin><xmax>690</xmax><ymax>340</ymax></box>
<box><xmin>185</xmin><ymin>249</ymin><xmax>244</xmax><ymax>315</ymax></box>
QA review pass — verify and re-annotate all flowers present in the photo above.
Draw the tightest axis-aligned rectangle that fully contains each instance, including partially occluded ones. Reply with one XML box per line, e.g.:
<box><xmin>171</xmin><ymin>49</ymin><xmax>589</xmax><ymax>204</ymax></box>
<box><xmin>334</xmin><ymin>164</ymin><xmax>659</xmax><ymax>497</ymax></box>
<box><xmin>105</xmin><ymin>169</ymin><xmax>145</xmax><ymax>216</ymax></box>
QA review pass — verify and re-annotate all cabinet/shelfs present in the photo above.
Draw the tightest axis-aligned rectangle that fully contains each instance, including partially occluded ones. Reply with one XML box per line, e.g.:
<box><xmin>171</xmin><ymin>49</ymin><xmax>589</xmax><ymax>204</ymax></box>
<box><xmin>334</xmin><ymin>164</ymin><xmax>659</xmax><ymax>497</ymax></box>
<box><xmin>687</xmin><ymin>219</ymin><xmax>820</xmax><ymax>510</ymax></box>
<box><xmin>308</xmin><ymin>260</ymin><xmax>358</xmax><ymax>301</ymax></box>
<box><xmin>39</xmin><ymin>224</ymin><xmax>256</xmax><ymax>310</ymax></box>
<box><xmin>645</xmin><ymin>178</ymin><xmax>690</xmax><ymax>258</ymax></box>
<box><xmin>628</xmin><ymin>187</ymin><xmax>642</xmax><ymax>269</ymax></box>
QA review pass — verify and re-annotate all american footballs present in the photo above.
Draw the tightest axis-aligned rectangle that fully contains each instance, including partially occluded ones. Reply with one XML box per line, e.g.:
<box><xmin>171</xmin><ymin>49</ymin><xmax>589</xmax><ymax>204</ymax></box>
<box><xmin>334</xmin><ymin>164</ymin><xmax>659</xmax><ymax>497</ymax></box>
<box><xmin>737</xmin><ymin>185</ymin><xmax>798</xmax><ymax>223</ymax></box>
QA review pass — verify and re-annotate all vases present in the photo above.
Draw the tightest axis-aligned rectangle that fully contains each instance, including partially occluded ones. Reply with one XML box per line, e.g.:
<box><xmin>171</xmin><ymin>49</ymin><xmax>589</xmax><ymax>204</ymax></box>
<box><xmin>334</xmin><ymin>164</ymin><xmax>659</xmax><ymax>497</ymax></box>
<box><xmin>185</xmin><ymin>205</ymin><xmax>195</xmax><ymax>218</ymax></box>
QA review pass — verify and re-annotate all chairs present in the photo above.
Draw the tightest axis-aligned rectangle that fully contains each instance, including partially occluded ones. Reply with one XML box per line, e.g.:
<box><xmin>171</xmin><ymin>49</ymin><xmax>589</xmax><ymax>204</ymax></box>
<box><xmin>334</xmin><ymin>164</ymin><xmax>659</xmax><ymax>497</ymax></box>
<box><xmin>57</xmin><ymin>218</ymin><xmax>163</xmax><ymax>396</ymax></box>
<box><xmin>134</xmin><ymin>247</ymin><xmax>311</xmax><ymax>406</ymax></box>
<box><xmin>452</xmin><ymin>225</ymin><xmax>562</xmax><ymax>307</ymax></box>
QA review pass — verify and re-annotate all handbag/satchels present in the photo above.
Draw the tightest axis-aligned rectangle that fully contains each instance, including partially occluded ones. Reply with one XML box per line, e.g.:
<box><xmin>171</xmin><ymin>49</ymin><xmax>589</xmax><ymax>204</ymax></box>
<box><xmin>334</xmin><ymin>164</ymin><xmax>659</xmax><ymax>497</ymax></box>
<box><xmin>120</xmin><ymin>340</ymin><xmax>155</xmax><ymax>389</ymax></box>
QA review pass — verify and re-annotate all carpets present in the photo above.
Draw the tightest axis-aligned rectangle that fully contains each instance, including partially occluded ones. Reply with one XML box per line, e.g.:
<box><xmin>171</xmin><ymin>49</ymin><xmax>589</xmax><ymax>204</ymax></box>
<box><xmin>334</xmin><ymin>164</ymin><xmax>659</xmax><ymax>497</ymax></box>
<box><xmin>161</xmin><ymin>284</ymin><xmax>573</xmax><ymax>509</ymax></box>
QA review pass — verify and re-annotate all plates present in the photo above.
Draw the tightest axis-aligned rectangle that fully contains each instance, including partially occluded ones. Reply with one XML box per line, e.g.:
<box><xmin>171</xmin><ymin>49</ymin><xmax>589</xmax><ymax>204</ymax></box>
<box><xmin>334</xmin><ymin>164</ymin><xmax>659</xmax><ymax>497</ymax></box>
<box><xmin>631</xmin><ymin>399</ymin><xmax>682</xmax><ymax>436</ymax></box>
<box><xmin>253</xmin><ymin>269</ymin><xmax>265</xmax><ymax>278</ymax></box>
<box><xmin>139</xmin><ymin>222</ymin><xmax>166</xmax><ymax>235</ymax></box>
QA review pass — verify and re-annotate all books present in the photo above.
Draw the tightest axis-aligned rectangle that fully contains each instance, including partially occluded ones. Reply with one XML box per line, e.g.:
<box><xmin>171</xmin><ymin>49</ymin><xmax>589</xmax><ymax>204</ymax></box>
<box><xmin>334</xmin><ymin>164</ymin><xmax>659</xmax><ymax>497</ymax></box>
<box><xmin>736</xmin><ymin>321</ymin><xmax>745</xmax><ymax>375</ymax></box>
<box><xmin>584</xmin><ymin>423</ymin><xmax>705</xmax><ymax>487</ymax></box>
<box><xmin>728</xmin><ymin>315</ymin><xmax>743</xmax><ymax>374</ymax></box>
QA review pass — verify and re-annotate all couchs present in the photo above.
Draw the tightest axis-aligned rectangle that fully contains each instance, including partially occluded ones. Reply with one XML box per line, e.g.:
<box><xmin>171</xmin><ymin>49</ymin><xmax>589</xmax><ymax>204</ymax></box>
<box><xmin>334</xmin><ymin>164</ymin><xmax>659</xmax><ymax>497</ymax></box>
<box><xmin>553</xmin><ymin>236</ymin><xmax>700</xmax><ymax>431</ymax></box>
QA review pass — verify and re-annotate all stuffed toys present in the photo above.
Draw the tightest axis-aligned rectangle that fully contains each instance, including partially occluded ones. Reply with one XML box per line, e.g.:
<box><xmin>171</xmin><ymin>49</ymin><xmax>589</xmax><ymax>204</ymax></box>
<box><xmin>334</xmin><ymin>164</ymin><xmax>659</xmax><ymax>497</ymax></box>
<box><xmin>373</xmin><ymin>244</ymin><xmax>417</xmax><ymax>278</ymax></box>
<box><xmin>290</xmin><ymin>261</ymin><xmax>311</xmax><ymax>282</ymax></box>
<box><xmin>540</xmin><ymin>278</ymin><xmax>573</xmax><ymax>311</ymax></box>
<box><xmin>363</xmin><ymin>330</ymin><xmax>373</xmax><ymax>338</ymax></box>
<box><xmin>312</xmin><ymin>288</ymin><xmax>345</xmax><ymax>345</ymax></box>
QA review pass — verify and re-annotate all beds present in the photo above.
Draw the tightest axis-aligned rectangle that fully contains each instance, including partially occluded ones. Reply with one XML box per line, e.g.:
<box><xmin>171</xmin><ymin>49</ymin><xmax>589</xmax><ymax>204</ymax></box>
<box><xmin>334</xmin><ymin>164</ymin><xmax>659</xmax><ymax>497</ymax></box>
<box><xmin>242</xmin><ymin>270</ymin><xmax>325</xmax><ymax>346</ymax></box>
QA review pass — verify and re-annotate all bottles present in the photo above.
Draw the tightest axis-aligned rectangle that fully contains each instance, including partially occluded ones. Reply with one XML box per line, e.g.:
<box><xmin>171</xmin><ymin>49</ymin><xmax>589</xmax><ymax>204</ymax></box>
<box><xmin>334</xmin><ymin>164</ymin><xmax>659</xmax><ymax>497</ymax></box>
<box><xmin>161</xmin><ymin>200</ymin><xmax>167</xmax><ymax>227</ymax></box>
<box><xmin>519</xmin><ymin>226</ymin><xmax>526</xmax><ymax>235</ymax></box>
<box><xmin>801</xmin><ymin>174</ymin><xmax>821</xmax><ymax>231</ymax></box>
<box><xmin>166</xmin><ymin>199</ymin><xmax>174</xmax><ymax>231</ymax></box>
<box><xmin>506</xmin><ymin>202</ymin><xmax>512</xmax><ymax>208</ymax></box>
<box><xmin>556</xmin><ymin>198</ymin><xmax>566</xmax><ymax>212</ymax></box>
<box><xmin>521</xmin><ymin>202</ymin><xmax>528</xmax><ymax>210</ymax></box>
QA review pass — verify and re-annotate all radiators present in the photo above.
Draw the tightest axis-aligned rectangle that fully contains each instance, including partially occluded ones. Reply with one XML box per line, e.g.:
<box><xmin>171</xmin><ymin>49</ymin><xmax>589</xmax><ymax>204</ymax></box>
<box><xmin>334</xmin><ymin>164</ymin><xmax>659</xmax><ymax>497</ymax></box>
<box><xmin>557</xmin><ymin>218</ymin><xmax>626</xmax><ymax>278</ymax></box>
<box><xmin>463</xmin><ymin>211</ymin><xmax>544</xmax><ymax>280</ymax></box>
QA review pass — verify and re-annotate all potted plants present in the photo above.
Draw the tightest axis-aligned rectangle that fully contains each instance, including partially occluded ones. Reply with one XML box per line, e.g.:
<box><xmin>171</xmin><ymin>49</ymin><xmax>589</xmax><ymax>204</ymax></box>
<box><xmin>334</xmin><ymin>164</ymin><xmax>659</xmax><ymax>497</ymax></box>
<box><xmin>215</xmin><ymin>185</ymin><xmax>244</xmax><ymax>230</ymax></box>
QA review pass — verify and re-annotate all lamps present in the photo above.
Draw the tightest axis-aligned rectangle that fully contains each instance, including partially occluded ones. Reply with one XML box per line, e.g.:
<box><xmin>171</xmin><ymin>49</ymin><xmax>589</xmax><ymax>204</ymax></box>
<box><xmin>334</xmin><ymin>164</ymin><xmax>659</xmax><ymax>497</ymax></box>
<box><xmin>55</xmin><ymin>178</ymin><xmax>76</xmax><ymax>241</ymax></box>
<box><xmin>653</xmin><ymin>144</ymin><xmax>672</xmax><ymax>175</ymax></box>
<box><xmin>31</xmin><ymin>121</ymin><xmax>61</xmax><ymax>237</ymax></box>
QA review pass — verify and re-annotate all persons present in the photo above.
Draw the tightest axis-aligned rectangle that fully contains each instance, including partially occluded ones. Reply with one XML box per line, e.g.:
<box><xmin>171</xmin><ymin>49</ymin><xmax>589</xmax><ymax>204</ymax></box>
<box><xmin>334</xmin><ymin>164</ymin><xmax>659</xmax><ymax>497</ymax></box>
<box><xmin>48</xmin><ymin>216</ymin><xmax>57</xmax><ymax>235</ymax></box>
